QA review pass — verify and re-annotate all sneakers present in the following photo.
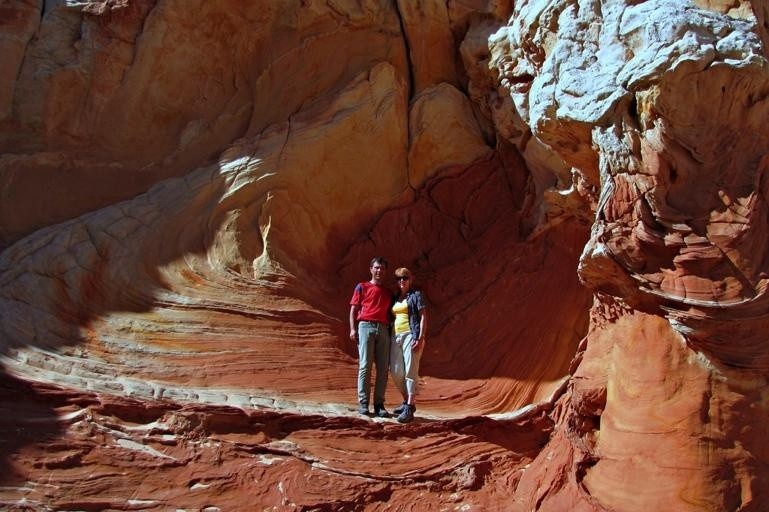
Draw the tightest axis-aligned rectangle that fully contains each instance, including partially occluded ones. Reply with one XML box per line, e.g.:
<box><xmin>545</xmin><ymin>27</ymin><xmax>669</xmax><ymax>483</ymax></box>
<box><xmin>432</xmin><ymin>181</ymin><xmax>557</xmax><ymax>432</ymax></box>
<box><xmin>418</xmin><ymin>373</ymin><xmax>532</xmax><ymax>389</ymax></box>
<box><xmin>374</xmin><ymin>404</ymin><xmax>389</xmax><ymax>417</ymax></box>
<box><xmin>394</xmin><ymin>402</ymin><xmax>416</xmax><ymax>423</ymax></box>
<box><xmin>359</xmin><ymin>402</ymin><xmax>370</xmax><ymax>416</ymax></box>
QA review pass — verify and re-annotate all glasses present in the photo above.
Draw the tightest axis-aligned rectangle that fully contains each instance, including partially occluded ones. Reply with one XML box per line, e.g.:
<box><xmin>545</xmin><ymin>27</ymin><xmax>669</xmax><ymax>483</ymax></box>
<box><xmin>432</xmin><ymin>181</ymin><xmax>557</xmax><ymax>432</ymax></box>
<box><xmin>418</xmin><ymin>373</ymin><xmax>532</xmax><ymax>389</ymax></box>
<box><xmin>396</xmin><ymin>275</ymin><xmax>409</xmax><ymax>282</ymax></box>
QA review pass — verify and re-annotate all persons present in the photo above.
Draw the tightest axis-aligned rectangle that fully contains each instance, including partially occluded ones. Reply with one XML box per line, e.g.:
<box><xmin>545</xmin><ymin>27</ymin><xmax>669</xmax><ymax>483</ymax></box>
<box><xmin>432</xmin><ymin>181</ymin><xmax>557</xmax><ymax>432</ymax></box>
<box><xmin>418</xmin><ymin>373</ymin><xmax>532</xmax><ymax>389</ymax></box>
<box><xmin>350</xmin><ymin>257</ymin><xmax>395</xmax><ymax>417</ymax></box>
<box><xmin>390</xmin><ymin>267</ymin><xmax>427</xmax><ymax>422</ymax></box>
